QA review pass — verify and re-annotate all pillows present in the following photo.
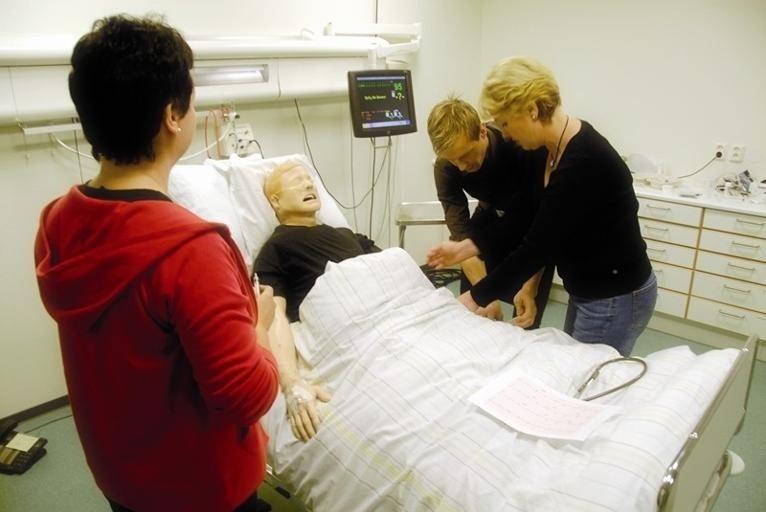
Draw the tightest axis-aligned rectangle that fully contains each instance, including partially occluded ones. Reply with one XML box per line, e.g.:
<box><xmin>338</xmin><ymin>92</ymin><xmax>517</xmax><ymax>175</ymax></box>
<box><xmin>225</xmin><ymin>154</ymin><xmax>342</xmax><ymax>257</ymax></box>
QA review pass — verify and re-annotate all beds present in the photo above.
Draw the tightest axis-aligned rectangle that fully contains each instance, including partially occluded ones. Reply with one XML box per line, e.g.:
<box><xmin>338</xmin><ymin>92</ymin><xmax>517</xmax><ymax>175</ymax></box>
<box><xmin>168</xmin><ymin>152</ymin><xmax>758</xmax><ymax>512</ymax></box>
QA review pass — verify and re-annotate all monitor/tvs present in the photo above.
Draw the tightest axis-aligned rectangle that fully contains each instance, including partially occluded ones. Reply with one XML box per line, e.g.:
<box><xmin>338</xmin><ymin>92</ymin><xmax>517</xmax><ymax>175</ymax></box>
<box><xmin>347</xmin><ymin>69</ymin><xmax>418</xmax><ymax>139</ymax></box>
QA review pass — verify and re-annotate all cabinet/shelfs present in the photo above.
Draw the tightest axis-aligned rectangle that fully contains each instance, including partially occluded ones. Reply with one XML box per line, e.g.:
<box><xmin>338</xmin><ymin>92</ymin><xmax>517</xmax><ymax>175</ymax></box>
<box><xmin>634</xmin><ymin>189</ymin><xmax>765</xmax><ymax>363</ymax></box>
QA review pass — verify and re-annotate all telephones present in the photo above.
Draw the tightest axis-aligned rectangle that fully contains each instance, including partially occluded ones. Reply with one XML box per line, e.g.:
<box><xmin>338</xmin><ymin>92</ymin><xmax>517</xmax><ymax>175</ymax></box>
<box><xmin>0</xmin><ymin>419</ymin><xmax>48</xmax><ymax>474</ymax></box>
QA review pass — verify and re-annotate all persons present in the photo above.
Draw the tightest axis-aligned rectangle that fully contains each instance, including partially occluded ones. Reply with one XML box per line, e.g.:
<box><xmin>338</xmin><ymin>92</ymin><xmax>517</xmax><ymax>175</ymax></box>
<box><xmin>428</xmin><ymin>91</ymin><xmax>559</xmax><ymax>333</ymax></box>
<box><xmin>238</xmin><ymin>159</ymin><xmax>390</xmax><ymax>444</ymax></box>
<box><xmin>431</xmin><ymin>54</ymin><xmax>658</xmax><ymax>357</ymax></box>
<box><xmin>30</xmin><ymin>7</ymin><xmax>280</xmax><ymax>511</ymax></box>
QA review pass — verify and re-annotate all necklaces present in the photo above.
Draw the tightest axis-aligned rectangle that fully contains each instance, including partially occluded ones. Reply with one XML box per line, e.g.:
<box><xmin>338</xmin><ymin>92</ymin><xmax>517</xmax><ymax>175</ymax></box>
<box><xmin>549</xmin><ymin>114</ymin><xmax>570</xmax><ymax>169</ymax></box>
